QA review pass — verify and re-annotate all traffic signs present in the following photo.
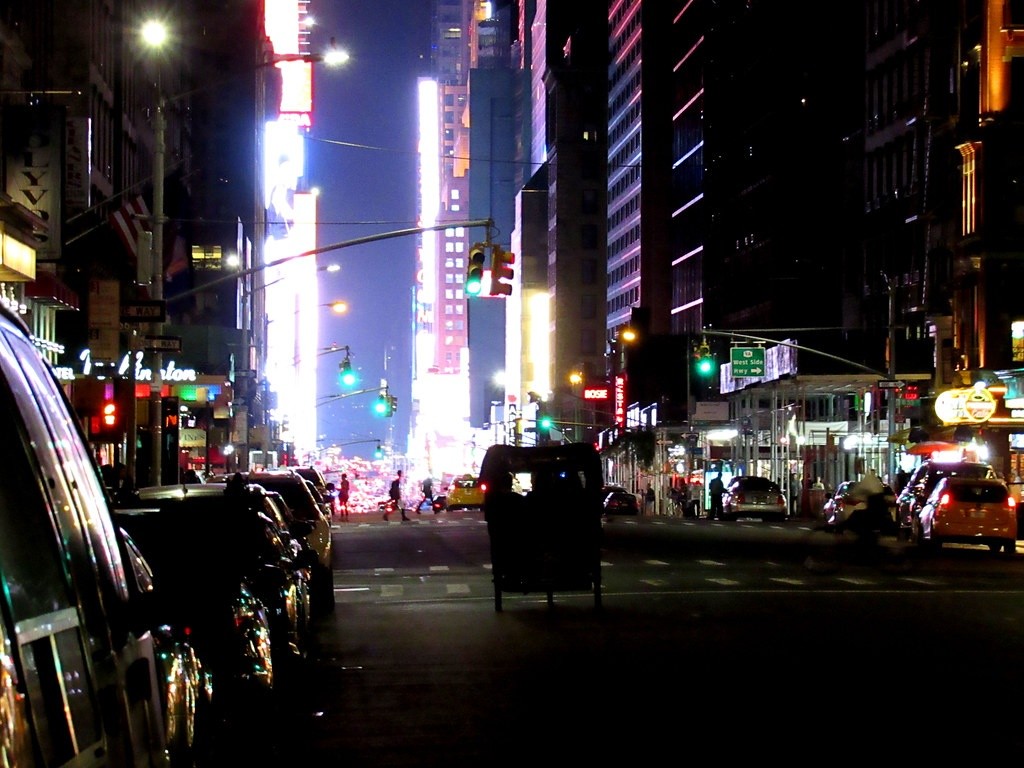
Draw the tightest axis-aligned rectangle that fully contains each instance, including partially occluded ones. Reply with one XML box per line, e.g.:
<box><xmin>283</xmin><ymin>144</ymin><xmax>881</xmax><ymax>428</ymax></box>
<box><xmin>730</xmin><ymin>347</ymin><xmax>764</xmax><ymax>377</ymax></box>
<box><xmin>877</xmin><ymin>380</ymin><xmax>906</xmax><ymax>390</ymax></box>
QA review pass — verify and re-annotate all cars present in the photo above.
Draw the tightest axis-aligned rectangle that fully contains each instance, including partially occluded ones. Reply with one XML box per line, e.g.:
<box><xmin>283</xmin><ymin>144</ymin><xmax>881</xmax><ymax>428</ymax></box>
<box><xmin>442</xmin><ymin>475</ymin><xmax>485</xmax><ymax>511</ymax></box>
<box><xmin>602</xmin><ymin>492</ymin><xmax>637</xmax><ymax>514</ymax></box>
<box><xmin>600</xmin><ymin>486</ymin><xmax>628</xmax><ymax>497</ymax></box>
<box><xmin>108</xmin><ymin>465</ymin><xmax>335</xmax><ymax>702</ymax></box>
<box><xmin>722</xmin><ymin>475</ymin><xmax>785</xmax><ymax>522</ymax></box>
<box><xmin>918</xmin><ymin>476</ymin><xmax>1018</xmax><ymax>552</ymax></box>
<box><xmin>822</xmin><ymin>481</ymin><xmax>900</xmax><ymax>531</ymax></box>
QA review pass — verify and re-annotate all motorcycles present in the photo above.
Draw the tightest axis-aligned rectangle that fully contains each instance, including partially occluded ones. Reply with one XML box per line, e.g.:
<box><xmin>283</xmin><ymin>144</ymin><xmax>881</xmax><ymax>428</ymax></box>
<box><xmin>483</xmin><ymin>442</ymin><xmax>607</xmax><ymax>612</ymax></box>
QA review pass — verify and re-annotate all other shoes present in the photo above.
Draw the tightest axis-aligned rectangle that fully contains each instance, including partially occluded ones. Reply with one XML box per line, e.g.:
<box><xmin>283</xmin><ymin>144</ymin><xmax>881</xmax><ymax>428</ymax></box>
<box><xmin>402</xmin><ymin>517</ymin><xmax>409</xmax><ymax>521</ymax></box>
<box><xmin>415</xmin><ymin>509</ymin><xmax>421</xmax><ymax>514</ymax></box>
<box><xmin>383</xmin><ymin>514</ymin><xmax>389</xmax><ymax>521</ymax></box>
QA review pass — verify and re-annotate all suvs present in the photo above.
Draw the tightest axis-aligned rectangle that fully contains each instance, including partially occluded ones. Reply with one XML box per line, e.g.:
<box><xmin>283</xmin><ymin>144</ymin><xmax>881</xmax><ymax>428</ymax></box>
<box><xmin>0</xmin><ymin>301</ymin><xmax>185</xmax><ymax>768</ymax></box>
<box><xmin>890</xmin><ymin>460</ymin><xmax>998</xmax><ymax>540</ymax></box>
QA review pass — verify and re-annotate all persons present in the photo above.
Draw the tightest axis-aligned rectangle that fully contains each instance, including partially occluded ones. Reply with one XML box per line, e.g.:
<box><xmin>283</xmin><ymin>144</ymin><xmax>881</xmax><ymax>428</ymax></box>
<box><xmin>336</xmin><ymin>473</ymin><xmax>349</xmax><ymax>517</ymax></box>
<box><xmin>644</xmin><ymin>471</ymin><xmax>824</xmax><ymax>520</ymax></box>
<box><xmin>416</xmin><ymin>476</ymin><xmax>437</xmax><ymax>515</ymax></box>
<box><xmin>383</xmin><ymin>470</ymin><xmax>410</xmax><ymax>521</ymax></box>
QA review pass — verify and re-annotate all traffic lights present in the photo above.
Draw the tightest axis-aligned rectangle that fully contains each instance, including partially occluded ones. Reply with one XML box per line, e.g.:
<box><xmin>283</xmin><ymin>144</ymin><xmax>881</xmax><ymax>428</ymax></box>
<box><xmin>699</xmin><ymin>346</ymin><xmax>712</xmax><ymax>375</ymax></box>
<box><xmin>491</xmin><ymin>251</ymin><xmax>513</xmax><ymax>297</ymax></box>
<box><xmin>343</xmin><ymin>360</ymin><xmax>354</xmax><ymax>384</ymax></box>
<box><xmin>390</xmin><ymin>395</ymin><xmax>398</xmax><ymax>415</ymax></box>
<box><xmin>542</xmin><ymin>413</ymin><xmax>551</xmax><ymax>440</ymax></box>
<box><xmin>374</xmin><ymin>394</ymin><xmax>389</xmax><ymax>416</ymax></box>
<box><xmin>466</xmin><ymin>248</ymin><xmax>484</xmax><ymax>294</ymax></box>
<box><xmin>376</xmin><ymin>448</ymin><xmax>384</xmax><ymax>460</ymax></box>
<box><xmin>338</xmin><ymin>364</ymin><xmax>348</xmax><ymax>386</ymax></box>
